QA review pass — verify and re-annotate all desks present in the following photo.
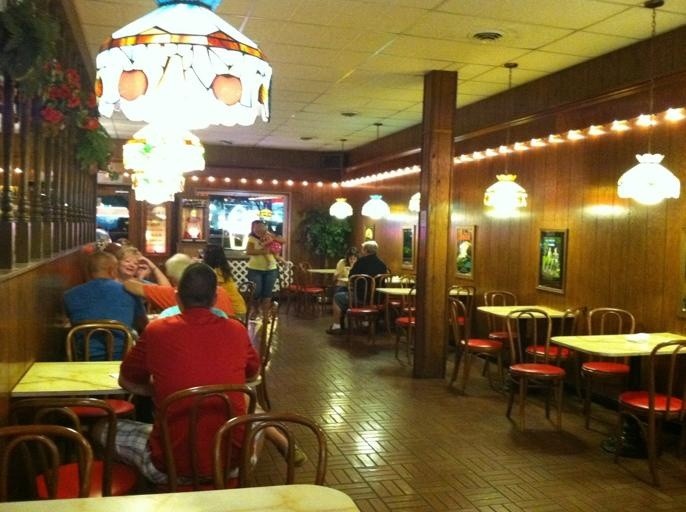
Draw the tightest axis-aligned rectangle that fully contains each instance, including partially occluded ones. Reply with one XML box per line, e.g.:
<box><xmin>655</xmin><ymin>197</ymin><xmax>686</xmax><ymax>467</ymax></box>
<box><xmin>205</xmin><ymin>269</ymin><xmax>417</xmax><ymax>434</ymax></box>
<box><xmin>10</xmin><ymin>360</ymin><xmax>262</xmax><ymax>422</ymax></box>
<box><xmin>49</xmin><ymin>311</ymin><xmax>159</xmax><ymax>329</ymax></box>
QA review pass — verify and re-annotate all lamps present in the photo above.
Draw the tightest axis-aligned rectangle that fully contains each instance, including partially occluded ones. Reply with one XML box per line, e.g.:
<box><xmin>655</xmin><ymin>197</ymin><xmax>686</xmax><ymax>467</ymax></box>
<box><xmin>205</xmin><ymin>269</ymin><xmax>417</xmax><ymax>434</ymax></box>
<box><xmin>93</xmin><ymin>0</ymin><xmax>271</xmax><ymax>130</ymax></box>
<box><xmin>618</xmin><ymin>0</ymin><xmax>683</xmax><ymax>205</ymax></box>
<box><xmin>121</xmin><ymin>123</ymin><xmax>206</xmax><ymax>206</ymax></box>
<box><xmin>329</xmin><ymin>61</ymin><xmax>530</xmax><ymax>229</ymax></box>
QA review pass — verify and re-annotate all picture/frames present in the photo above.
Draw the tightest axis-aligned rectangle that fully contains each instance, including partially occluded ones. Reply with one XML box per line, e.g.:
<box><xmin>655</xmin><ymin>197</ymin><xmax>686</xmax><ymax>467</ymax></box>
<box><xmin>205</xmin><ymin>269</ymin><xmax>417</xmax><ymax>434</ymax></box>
<box><xmin>399</xmin><ymin>223</ymin><xmax>417</xmax><ymax>271</ymax></box>
<box><xmin>536</xmin><ymin>227</ymin><xmax>567</xmax><ymax>293</ymax></box>
<box><xmin>453</xmin><ymin>226</ymin><xmax>477</xmax><ymax>281</ymax></box>
<box><xmin>676</xmin><ymin>243</ymin><xmax>686</xmax><ymax>320</ymax></box>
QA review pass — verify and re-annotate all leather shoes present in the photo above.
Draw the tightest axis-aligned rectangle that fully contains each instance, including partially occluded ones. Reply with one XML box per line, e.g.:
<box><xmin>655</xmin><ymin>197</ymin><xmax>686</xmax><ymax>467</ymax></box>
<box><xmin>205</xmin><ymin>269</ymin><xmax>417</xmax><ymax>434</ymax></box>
<box><xmin>278</xmin><ymin>444</ymin><xmax>307</xmax><ymax>466</ymax></box>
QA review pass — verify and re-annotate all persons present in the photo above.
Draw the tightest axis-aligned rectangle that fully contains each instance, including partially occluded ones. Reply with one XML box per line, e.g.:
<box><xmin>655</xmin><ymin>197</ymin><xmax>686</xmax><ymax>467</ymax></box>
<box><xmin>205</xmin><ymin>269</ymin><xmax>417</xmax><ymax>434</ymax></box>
<box><xmin>246</xmin><ymin>221</ymin><xmax>287</xmax><ymax>324</ymax></box>
<box><xmin>160</xmin><ymin>302</ymin><xmax>306</xmax><ymax>467</ymax></box>
<box><xmin>334</xmin><ymin>248</ymin><xmax>359</xmax><ymax>292</ymax></box>
<box><xmin>325</xmin><ymin>239</ymin><xmax>387</xmax><ymax>334</ymax></box>
<box><xmin>63</xmin><ymin>229</ymin><xmax>248</xmax><ymax>362</ymax></box>
<box><xmin>93</xmin><ymin>263</ymin><xmax>259</xmax><ymax>484</ymax></box>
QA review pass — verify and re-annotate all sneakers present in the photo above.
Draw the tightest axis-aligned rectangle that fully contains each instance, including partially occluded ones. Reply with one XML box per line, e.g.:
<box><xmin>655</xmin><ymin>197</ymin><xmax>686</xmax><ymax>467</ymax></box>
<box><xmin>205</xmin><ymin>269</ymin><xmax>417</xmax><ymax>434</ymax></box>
<box><xmin>354</xmin><ymin>325</ymin><xmax>369</xmax><ymax>335</ymax></box>
<box><xmin>326</xmin><ymin>326</ymin><xmax>344</xmax><ymax>335</ymax></box>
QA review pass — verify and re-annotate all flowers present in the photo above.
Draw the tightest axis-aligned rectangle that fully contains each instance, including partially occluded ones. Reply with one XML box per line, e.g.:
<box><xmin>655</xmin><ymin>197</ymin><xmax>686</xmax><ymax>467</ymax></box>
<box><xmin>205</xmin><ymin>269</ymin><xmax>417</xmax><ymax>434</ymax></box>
<box><xmin>32</xmin><ymin>55</ymin><xmax>99</xmax><ymax>138</ymax></box>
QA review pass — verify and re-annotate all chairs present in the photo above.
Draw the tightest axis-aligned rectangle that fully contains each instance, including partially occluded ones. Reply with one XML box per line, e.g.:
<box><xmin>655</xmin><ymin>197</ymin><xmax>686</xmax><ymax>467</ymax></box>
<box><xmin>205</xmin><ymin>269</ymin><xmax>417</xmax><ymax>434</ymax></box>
<box><xmin>11</xmin><ymin>398</ymin><xmax>138</xmax><ymax>496</ymax></box>
<box><xmin>213</xmin><ymin>408</ymin><xmax>328</xmax><ymax>491</ymax></box>
<box><xmin>242</xmin><ymin>298</ymin><xmax>280</xmax><ymax>409</ymax></box>
<box><xmin>2</xmin><ymin>424</ymin><xmax>93</xmax><ymax>499</ymax></box>
<box><xmin>236</xmin><ymin>281</ymin><xmax>256</xmax><ymax>324</ymax></box>
<box><xmin>64</xmin><ymin>322</ymin><xmax>138</xmax><ymax>425</ymax></box>
<box><xmin>342</xmin><ymin>257</ymin><xmax>503</xmax><ymax>390</ymax></box>
<box><xmin>69</xmin><ymin>317</ymin><xmax>140</xmax><ymax>347</ymax></box>
<box><xmin>280</xmin><ymin>260</ymin><xmax>342</xmax><ymax>326</ymax></box>
<box><xmin>158</xmin><ymin>384</ymin><xmax>257</xmax><ymax>491</ymax></box>
<box><xmin>504</xmin><ymin>290</ymin><xmax>686</xmax><ymax>482</ymax></box>
